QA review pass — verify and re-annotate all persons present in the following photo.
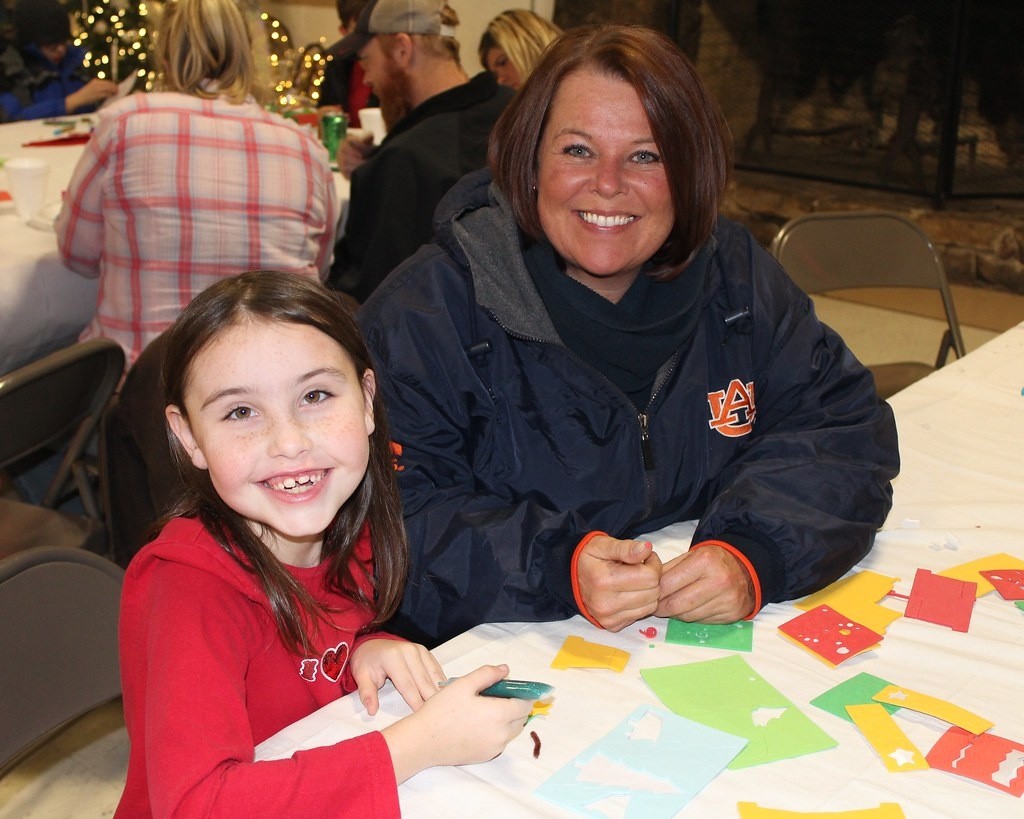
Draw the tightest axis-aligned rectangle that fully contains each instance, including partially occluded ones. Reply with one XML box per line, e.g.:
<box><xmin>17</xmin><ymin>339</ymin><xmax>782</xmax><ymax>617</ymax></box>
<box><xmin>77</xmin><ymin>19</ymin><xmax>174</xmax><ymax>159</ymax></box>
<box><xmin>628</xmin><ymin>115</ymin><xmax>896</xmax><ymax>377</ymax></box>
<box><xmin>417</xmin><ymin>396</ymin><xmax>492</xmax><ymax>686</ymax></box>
<box><xmin>0</xmin><ymin>0</ymin><xmax>572</xmax><ymax>127</ymax></box>
<box><xmin>356</xmin><ymin>27</ymin><xmax>905</xmax><ymax>652</ymax></box>
<box><xmin>323</xmin><ymin>1</ymin><xmax>518</xmax><ymax>306</ymax></box>
<box><xmin>104</xmin><ymin>262</ymin><xmax>539</xmax><ymax>818</ymax></box>
<box><xmin>55</xmin><ymin>1</ymin><xmax>347</xmax><ymax>572</ymax></box>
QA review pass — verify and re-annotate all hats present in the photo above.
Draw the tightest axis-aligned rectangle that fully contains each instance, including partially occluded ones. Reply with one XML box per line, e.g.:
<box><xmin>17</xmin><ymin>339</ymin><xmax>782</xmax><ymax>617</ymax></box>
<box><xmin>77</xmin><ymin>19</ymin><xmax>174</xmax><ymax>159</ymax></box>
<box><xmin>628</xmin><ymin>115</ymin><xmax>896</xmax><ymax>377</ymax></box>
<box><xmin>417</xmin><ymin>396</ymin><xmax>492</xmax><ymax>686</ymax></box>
<box><xmin>15</xmin><ymin>0</ymin><xmax>70</xmax><ymax>51</ymax></box>
<box><xmin>325</xmin><ymin>0</ymin><xmax>457</xmax><ymax>60</ymax></box>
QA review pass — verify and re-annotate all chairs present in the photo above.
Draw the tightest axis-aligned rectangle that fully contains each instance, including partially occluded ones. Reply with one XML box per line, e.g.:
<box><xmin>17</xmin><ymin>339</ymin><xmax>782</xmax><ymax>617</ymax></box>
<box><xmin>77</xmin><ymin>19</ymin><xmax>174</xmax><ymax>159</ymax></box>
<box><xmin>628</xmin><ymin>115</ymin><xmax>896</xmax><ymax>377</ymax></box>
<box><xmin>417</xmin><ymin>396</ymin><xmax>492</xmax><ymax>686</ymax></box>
<box><xmin>771</xmin><ymin>210</ymin><xmax>967</xmax><ymax>403</ymax></box>
<box><xmin>0</xmin><ymin>542</ymin><xmax>140</xmax><ymax>819</ymax></box>
<box><xmin>0</xmin><ymin>336</ymin><xmax>127</xmax><ymax>567</ymax></box>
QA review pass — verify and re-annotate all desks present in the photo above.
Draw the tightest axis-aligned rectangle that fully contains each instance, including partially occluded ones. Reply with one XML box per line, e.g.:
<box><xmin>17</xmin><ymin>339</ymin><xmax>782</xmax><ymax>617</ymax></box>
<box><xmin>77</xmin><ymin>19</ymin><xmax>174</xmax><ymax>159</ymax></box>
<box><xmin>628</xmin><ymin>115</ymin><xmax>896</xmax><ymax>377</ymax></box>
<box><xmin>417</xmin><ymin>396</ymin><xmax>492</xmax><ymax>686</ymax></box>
<box><xmin>0</xmin><ymin>107</ymin><xmax>387</xmax><ymax>374</ymax></box>
<box><xmin>253</xmin><ymin>325</ymin><xmax>1024</xmax><ymax>819</ymax></box>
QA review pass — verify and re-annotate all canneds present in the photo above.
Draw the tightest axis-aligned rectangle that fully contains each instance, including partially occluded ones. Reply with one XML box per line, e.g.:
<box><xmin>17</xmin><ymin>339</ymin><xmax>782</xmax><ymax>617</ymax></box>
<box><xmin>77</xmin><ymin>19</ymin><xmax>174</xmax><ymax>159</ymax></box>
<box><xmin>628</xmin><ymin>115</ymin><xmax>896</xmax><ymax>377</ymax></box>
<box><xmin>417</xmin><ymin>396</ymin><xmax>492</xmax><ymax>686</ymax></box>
<box><xmin>321</xmin><ymin>112</ymin><xmax>348</xmax><ymax>172</ymax></box>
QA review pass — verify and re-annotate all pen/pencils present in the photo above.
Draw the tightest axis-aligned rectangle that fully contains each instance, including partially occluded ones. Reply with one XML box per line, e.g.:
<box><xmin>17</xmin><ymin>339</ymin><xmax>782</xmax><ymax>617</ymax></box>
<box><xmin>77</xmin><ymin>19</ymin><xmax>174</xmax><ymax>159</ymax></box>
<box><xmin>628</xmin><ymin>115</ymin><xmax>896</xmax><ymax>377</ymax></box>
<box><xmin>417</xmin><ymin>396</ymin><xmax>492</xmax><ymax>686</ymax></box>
<box><xmin>45</xmin><ymin>118</ymin><xmax>94</xmax><ymax>134</ymax></box>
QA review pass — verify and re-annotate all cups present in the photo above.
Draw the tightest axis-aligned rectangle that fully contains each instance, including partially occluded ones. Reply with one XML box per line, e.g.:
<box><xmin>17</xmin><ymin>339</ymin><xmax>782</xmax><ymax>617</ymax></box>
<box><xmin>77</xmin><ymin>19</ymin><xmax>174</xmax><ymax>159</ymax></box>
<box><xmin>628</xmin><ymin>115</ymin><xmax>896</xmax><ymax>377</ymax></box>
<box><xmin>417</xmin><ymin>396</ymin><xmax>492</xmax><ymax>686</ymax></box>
<box><xmin>3</xmin><ymin>158</ymin><xmax>47</xmax><ymax>213</ymax></box>
